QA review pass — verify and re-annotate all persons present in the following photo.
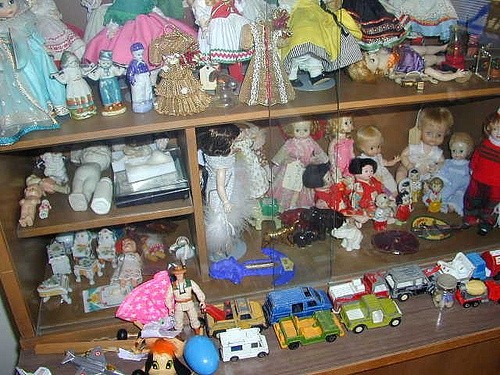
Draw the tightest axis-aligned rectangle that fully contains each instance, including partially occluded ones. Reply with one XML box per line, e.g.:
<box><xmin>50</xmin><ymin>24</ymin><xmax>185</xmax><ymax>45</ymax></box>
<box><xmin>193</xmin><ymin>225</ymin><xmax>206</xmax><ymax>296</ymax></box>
<box><xmin>0</xmin><ymin>0</ymin><xmax>500</xmax><ymax>375</ymax></box>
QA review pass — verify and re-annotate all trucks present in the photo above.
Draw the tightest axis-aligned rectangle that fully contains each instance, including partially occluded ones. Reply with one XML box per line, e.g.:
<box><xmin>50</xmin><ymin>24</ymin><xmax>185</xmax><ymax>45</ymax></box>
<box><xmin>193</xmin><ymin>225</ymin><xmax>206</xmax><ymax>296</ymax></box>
<box><xmin>218</xmin><ymin>327</ymin><xmax>270</xmax><ymax>362</ymax></box>
<box><xmin>328</xmin><ymin>272</ymin><xmax>391</xmax><ymax>310</ymax></box>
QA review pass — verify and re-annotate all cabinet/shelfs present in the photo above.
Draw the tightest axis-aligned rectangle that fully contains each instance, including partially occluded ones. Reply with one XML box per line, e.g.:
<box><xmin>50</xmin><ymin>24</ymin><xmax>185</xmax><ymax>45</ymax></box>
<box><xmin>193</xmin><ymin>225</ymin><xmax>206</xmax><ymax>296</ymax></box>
<box><xmin>0</xmin><ymin>57</ymin><xmax>499</xmax><ymax>375</ymax></box>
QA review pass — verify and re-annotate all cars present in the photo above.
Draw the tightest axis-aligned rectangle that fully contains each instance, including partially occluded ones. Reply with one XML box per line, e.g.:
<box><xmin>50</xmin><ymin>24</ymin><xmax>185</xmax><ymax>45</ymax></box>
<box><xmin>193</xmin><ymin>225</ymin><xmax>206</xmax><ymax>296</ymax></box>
<box><xmin>274</xmin><ymin>310</ymin><xmax>345</xmax><ymax>350</ymax></box>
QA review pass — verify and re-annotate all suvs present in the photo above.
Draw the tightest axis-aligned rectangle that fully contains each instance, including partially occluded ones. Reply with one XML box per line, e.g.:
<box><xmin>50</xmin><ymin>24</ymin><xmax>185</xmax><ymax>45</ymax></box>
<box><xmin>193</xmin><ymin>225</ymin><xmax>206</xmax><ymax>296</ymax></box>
<box><xmin>263</xmin><ymin>286</ymin><xmax>334</xmax><ymax>324</ymax></box>
<box><xmin>385</xmin><ymin>265</ymin><xmax>432</xmax><ymax>302</ymax></box>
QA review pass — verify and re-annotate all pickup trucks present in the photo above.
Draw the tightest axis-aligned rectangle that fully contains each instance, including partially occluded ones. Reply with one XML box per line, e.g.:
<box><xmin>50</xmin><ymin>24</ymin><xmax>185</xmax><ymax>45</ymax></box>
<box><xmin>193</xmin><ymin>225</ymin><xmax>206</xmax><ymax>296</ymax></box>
<box><xmin>339</xmin><ymin>293</ymin><xmax>403</xmax><ymax>334</ymax></box>
<box><xmin>205</xmin><ymin>297</ymin><xmax>268</xmax><ymax>338</ymax></box>
